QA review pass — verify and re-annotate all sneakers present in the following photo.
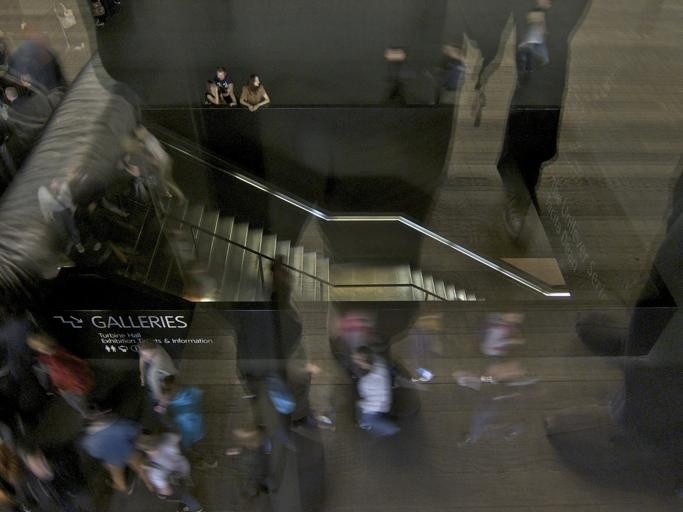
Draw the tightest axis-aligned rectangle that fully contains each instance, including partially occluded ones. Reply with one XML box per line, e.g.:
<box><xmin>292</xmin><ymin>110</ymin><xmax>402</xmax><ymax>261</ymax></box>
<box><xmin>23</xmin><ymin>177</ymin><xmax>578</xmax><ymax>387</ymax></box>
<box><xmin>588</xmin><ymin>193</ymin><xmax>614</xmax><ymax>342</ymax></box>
<box><xmin>180</xmin><ymin>505</ymin><xmax>203</xmax><ymax>512</ymax></box>
<box><xmin>195</xmin><ymin>459</ymin><xmax>218</xmax><ymax>471</ymax></box>
<box><xmin>318</xmin><ymin>416</ymin><xmax>336</xmax><ymax>432</ymax></box>
<box><xmin>232</xmin><ymin>426</ymin><xmax>257</xmax><ymax>437</ymax></box>
<box><xmin>412</xmin><ymin>371</ymin><xmax>431</xmax><ymax>383</ymax></box>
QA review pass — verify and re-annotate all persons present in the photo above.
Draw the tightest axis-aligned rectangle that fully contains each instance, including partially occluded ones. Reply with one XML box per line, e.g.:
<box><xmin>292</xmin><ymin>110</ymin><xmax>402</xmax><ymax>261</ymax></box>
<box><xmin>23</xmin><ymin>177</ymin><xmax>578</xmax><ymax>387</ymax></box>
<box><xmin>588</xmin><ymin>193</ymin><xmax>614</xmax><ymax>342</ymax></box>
<box><xmin>0</xmin><ymin>0</ymin><xmax>678</xmax><ymax>509</ymax></box>
